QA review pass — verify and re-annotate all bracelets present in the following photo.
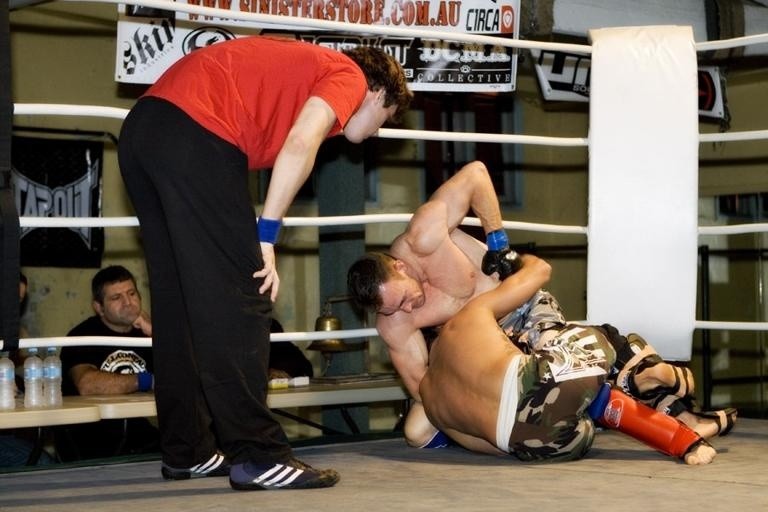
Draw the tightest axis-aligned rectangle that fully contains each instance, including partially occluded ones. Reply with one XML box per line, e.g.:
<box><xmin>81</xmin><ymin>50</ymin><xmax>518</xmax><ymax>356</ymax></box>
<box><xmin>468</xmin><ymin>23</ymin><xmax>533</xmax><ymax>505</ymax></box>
<box><xmin>125</xmin><ymin>374</ymin><xmax>129</xmax><ymax>393</ymax></box>
<box><xmin>486</xmin><ymin>228</ymin><xmax>509</xmax><ymax>252</ymax></box>
<box><xmin>257</xmin><ymin>215</ymin><xmax>282</xmax><ymax>244</ymax></box>
<box><xmin>137</xmin><ymin>371</ymin><xmax>152</xmax><ymax>392</ymax></box>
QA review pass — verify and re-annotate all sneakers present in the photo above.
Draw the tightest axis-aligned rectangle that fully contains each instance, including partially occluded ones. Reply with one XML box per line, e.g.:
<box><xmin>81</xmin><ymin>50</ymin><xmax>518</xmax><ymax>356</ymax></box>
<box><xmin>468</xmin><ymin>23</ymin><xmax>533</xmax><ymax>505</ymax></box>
<box><xmin>162</xmin><ymin>453</ymin><xmax>232</xmax><ymax>480</ymax></box>
<box><xmin>231</xmin><ymin>457</ymin><xmax>341</xmax><ymax>489</ymax></box>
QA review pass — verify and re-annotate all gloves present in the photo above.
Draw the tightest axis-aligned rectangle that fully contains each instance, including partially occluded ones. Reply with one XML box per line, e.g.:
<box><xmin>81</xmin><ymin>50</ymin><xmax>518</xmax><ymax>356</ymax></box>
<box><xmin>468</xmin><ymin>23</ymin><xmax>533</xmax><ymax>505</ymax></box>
<box><xmin>481</xmin><ymin>228</ymin><xmax>524</xmax><ymax>281</ymax></box>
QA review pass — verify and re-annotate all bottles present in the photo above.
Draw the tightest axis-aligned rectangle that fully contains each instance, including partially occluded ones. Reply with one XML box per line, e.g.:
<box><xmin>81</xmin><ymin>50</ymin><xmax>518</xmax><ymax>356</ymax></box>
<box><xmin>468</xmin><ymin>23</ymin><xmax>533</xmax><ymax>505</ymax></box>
<box><xmin>22</xmin><ymin>348</ymin><xmax>63</xmax><ymax>411</ymax></box>
<box><xmin>0</xmin><ymin>349</ymin><xmax>17</xmax><ymax>414</ymax></box>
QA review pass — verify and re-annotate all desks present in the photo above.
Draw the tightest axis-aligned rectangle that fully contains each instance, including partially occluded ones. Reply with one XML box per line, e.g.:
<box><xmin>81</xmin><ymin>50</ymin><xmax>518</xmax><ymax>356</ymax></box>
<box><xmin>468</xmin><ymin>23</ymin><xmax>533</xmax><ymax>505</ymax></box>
<box><xmin>0</xmin><ymin>372</ymin><xmax>412</xmax><ymax>469</ymax></box>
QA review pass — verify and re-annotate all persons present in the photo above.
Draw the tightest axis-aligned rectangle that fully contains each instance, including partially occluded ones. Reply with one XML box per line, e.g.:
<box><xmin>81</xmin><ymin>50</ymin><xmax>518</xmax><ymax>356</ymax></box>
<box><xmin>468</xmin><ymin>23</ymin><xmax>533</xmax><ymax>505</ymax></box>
<box><xmin>403</xmin><ymin>254</ymin><xmax>738</xmax><ymax>464</ymax></box>
<box><xmin>347</xmin><ymin>160</ymin><xmax>716</xmax><ymax>464</ymax></box>
<box><xmin>269</xmin><ymin>318</ymin><xmax>312</xmax><ymax>378</ymax></box>
<box><xmin>117</xmin><ymin>37</ymin><xmax>415</xmax><ymax>491</ymax></box>
<box><xmin>58</xmin><ymin>266</ymin><xmax>162</xmax><ymax>461</ymax></box>
<box><xmin>1</xmin><ymin>274</ymin><xmax>60</xmax><ymax>465</ymax></box>
